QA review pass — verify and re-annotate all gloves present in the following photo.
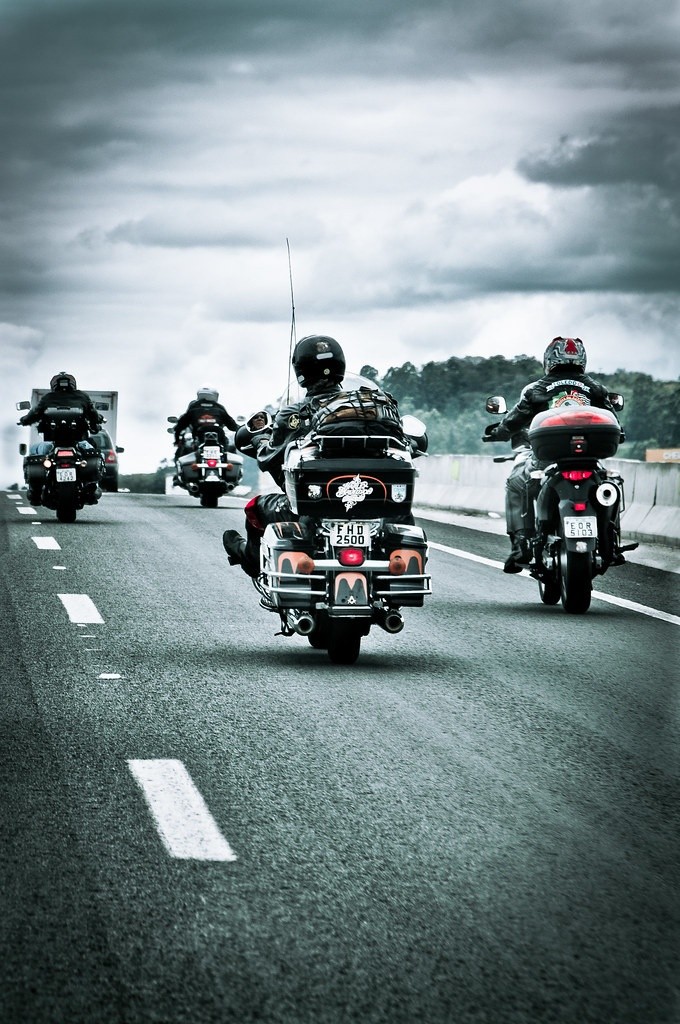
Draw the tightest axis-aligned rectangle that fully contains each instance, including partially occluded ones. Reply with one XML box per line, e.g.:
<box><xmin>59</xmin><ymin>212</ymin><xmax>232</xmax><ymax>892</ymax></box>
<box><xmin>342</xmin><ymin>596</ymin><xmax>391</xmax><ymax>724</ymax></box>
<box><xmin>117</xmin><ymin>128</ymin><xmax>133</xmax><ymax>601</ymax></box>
<box><xmin>250</xmin><ymin>432</ymin><xmax>271</xmax><ymax>447</ymax></box>
<box><xmin>490</xmin><ymin>423</ymin><xmax>511</xmax><ymax>442</ymax></box>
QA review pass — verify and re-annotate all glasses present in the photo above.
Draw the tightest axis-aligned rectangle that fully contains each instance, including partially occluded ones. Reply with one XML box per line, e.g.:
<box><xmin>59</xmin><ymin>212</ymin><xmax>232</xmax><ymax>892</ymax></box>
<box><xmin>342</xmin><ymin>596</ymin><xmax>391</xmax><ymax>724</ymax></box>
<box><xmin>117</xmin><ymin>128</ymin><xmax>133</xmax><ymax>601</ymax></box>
<box><xmin>252</xmin><ymin>414</ymin><xmax>266</xmax><ymax>419</ymax></box>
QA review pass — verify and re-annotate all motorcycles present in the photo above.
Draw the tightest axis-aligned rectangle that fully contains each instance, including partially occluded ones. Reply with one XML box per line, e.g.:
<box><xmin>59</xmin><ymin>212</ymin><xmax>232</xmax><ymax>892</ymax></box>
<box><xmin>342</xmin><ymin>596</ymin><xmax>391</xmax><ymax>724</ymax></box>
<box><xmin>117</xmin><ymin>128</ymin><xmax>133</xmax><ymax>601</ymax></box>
<box><xmin>166</xmin><ymin>415</ymin><xmax>247</xmax><ymax>509</ymax></box>
<box><xmin>482</xmin><ymin>390</ymin><xmax>641</xmax><ymax>615</ymax></box>
<box><xmin>227</xmin><ymin>373</ymin><xmax>430</xmax><ymax>664</ymax></box>
<box><xmin>15</xmin><ymin>402</ymin><xmax>126</xmax><ymax>525</ymax></box>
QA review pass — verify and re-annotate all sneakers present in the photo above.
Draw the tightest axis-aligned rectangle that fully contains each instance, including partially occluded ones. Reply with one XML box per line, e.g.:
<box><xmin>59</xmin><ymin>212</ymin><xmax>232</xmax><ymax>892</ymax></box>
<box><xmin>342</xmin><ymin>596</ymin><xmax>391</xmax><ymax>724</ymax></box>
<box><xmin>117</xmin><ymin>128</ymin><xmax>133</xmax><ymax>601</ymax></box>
<box><xmin>503</xmin><ymin>531</ymin><xmax>534</xmax><ymax>574</ymax></box>
<box><xmin>609</xmin><ymin>551</ymin><xmax>626</xmax><ymax>567</ymax></box>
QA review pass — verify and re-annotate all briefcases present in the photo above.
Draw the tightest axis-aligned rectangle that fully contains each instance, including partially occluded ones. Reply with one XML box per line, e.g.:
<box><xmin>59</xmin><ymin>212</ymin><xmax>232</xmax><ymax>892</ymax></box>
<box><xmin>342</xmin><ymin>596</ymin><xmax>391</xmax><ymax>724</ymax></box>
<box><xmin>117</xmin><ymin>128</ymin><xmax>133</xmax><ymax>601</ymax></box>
<box><xmin>43</xmin><ymin>405</ymin><xmax>85</xmax><ymax>442</ymax></box>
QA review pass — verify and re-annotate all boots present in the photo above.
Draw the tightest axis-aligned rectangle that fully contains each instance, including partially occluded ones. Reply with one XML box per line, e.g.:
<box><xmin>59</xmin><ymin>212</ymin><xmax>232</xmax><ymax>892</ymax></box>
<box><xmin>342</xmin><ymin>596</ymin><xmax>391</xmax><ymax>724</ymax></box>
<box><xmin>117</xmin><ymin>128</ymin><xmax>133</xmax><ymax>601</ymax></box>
<box><xmin>222</xmin><ymin>529</ymin><xmax>259</xmax><ymax>579</ymax></box>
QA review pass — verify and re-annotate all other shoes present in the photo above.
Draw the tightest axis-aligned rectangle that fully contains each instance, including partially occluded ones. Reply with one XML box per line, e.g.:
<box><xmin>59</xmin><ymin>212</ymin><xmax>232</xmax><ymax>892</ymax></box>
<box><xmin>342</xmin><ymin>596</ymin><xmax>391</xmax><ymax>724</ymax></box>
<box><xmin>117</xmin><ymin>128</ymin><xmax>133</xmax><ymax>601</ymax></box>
<box><xmin>26</xmin><ymin>489</ymin><xmax>41</xmax><ymax>507</ymax></box>
<box><xmin>85</xmin><ymin>484</ymin><xmax>98</xmax><ymax>505</ymax></box>
<box><xmin>173</xmin><ymin>478</ymin><xmax>183</xmax><ymax>486</ymax></box>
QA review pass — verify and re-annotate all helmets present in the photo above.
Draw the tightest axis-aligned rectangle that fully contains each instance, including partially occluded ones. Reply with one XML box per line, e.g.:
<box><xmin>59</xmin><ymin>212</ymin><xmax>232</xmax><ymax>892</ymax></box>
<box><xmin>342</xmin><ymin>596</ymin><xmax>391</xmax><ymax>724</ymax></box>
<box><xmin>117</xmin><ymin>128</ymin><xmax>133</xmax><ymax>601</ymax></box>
<box><xmin>291</xmin><ymin>333</ymin><xmax>347</xmax><ymax>389</ymax></box>
<box><xmin>543</xmin><ymin>336</ymin><xmax>587</xmax><ymax>376</ymax></box>
<box><xmin>197</xmin><ymin>383</ymin><xmax>219</xmax><ymax>402</ymax></box>
<box><xmin>50</xmin><ymin>371</ymin><xmax>77</xmax><ymax>392</ymax></box>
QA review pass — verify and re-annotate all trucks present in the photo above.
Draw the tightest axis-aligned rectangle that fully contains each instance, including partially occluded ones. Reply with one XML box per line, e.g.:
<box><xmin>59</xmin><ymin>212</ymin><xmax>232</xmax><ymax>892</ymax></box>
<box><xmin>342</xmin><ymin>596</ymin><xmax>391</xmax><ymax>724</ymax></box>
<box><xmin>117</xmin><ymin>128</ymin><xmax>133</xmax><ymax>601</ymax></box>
<box><xmin>30</xmin><ymin>388</ymin><xmax>119</xmax><ymax>492</ymax></box>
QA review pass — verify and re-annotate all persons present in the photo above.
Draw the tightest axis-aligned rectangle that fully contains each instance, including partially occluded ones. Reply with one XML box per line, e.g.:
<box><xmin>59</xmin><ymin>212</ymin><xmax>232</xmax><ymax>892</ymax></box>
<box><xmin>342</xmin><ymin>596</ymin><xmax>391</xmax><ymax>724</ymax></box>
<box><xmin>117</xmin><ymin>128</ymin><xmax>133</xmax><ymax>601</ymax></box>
<box><xmin>223</xmin><ymin>333</ymin><xmax>429</xmax><ymax>578</ymax></box>
<box><xmin>172</xmin><ymin>383</ymin><xmax>244</xmax><ymax>490</ymax></box>
<box><xmin>490</xmin><ymin>336</ymin><xmax>627</xmax><ymax>573</ymax></box>
<box><xmin>20</xmin><ymin>367</ymin><xmax>105</xmax><ymax>507</ymax></box>
<box><xmin>247</xmin><ymin>410</ymin><xmax>268</xmax><ymax>432</ymax></box>
